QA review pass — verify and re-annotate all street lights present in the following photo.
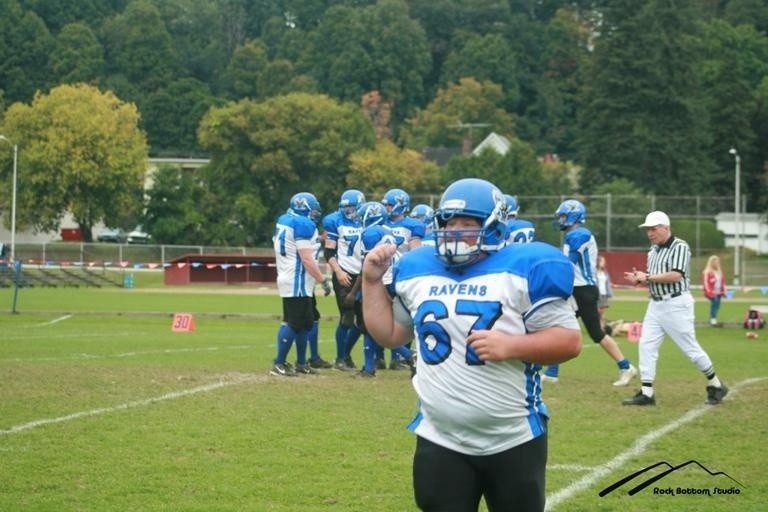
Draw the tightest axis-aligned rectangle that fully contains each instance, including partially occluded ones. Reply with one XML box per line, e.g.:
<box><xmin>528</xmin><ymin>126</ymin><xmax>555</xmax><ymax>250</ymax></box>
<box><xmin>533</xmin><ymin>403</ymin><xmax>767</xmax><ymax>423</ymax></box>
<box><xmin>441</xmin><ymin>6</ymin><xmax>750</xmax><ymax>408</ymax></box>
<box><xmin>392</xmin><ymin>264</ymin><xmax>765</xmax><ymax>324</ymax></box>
<box><xmin>1</xmin><ymin>134</ymin><xmax>18</xmax><ymax>267</ymax></box>
<box><xmin>729</xmin><ymin>146</ymin><xmax>741</xmax><ymax>284</ymax></box>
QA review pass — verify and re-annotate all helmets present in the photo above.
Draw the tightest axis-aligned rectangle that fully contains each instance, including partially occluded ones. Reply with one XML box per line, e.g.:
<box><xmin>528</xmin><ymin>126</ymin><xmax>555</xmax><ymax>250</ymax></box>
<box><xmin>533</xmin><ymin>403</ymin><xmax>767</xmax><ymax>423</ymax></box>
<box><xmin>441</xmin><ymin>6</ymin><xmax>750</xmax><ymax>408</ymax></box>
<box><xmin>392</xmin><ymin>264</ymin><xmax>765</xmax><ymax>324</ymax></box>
<box><xmin>380</xmin><ymin>188</ymin><xmax>411</xmax><ymax>218</ymax></box>
<box><xmin>552</xmin><ymin>200</ymin><xmax>587</xmax><ymax>233</ymax></box>
<box><xmin>504</xmin><ymin>194</ymin><xmax>520</xmax><ymax>218</ymax></box>
<box><xmin>430</xmin><ymin>177</ymin><xmax>508</xmax><ymax>269</ymax></box>
<box><xmin>287</xmin><ymin>192</ymin><xmax>323</xmax><ymax>226</ymax></box>
<box><xmin>410</xmin><ymin>204</ymin><xmax>436</xmax><ymax>229</ymax></box>
<box><xmin>338</xmin><ymin>190</ymin><xmax>367</xmax><ymax>220</ymax></box>
<box><xmin>356</xmin><ymin>201</ymin><xmax>389</xmax><ymax>229</ymax></box>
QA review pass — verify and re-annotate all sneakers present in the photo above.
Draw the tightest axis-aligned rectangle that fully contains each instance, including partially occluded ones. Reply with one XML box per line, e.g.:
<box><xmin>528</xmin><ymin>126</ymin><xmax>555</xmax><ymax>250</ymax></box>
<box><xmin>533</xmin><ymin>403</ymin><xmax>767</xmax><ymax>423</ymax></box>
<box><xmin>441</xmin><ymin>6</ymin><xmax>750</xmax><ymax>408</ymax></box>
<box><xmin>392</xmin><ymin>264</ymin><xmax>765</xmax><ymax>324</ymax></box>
<box><xmin>704</xmin><ymin>381</ymin><xmax>729</xmax><ymax>405</ymax></box>
<box><xmin>294</xmin><ymin>360</ymin><xmax>321</xmax><ymax>376</ymax></box>
<box><xmin>389</xmin><ymin>354</ymin><xmax>410</xmax><ymax>370</ymax></box>
<box><xmin>541</xmin><ymin>374</ymin><xmax>559</xmax><ymax>384</ymax></box>
<box><xmin>407</xmin><ymin>352</ymin><xmax>418</xmax><ymax>379</ymax></box>
<box><xmin>306</xmin><ymin>356</ymin><xmax>333</xmax><ymax>369</ymax></box>
<box><xmin>621</xmin><ymin>388</ymin><xmax>657</xmax><ymax>407</ymax></box>
<box><xmin>350</xmin><ymin>366</ymin><xmax>376</xmax><ymax>381</ymax></box>
<box><xmin>374</xmin><ymin>357</ymin><xmax>386</xmax><ymax>369</ymax></box>
<box><xmin>332</xmin><ymin>354</ymin><xmax>358</xmax><ymax>372</ymax></box>
<box><xmin>612</xmin><ymin>363</ymin><xmax>638</xmax><ymax>388</ymax></box>
<box><xmin>270</xmin><ymin>358</ymin><xmax>301</xmax><ymax>379</ymax></box>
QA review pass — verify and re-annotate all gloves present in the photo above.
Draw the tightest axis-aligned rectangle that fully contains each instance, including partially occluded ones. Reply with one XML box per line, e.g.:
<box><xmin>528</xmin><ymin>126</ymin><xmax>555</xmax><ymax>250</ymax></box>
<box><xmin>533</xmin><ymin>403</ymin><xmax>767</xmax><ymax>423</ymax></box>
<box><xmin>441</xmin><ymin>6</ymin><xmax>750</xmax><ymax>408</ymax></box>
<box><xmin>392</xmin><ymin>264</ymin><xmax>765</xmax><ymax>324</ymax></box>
<box><xmin>320</xmin><ymin>277</ymin><xmax>332</xmax><ymax>296</ymax></box>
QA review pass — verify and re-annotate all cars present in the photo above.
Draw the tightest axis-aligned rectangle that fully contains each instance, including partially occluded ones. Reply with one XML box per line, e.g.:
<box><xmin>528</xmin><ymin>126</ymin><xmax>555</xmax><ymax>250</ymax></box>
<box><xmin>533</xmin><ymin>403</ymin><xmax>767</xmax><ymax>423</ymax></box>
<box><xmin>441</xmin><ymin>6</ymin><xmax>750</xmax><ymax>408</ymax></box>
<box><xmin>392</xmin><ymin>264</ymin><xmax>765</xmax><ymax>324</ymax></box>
<box><xmin>128</xmin><ymin>224</ymin><xmax>152</xmax><ymax>244</ymax></box>
<box><xmin>98</xmin><ymin>228</ymin><xmax>128</xmax><ymax>243</ymax></box>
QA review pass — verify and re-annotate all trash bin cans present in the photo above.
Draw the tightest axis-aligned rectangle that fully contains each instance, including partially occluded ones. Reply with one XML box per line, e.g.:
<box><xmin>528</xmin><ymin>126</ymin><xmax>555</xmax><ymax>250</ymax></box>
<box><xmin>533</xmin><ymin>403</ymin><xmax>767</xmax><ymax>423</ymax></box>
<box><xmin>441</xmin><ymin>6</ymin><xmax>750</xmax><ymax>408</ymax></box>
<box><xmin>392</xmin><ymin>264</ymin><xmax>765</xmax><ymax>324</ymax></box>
<box><xmin>62</xmin><ymin>229</ymin><xmax>84</xmax><ymax>240</ymax></box>
<box><xmin>124</xmin><ymin>273</ymin><xmax>133</xmax><ymax>289</ymax></box>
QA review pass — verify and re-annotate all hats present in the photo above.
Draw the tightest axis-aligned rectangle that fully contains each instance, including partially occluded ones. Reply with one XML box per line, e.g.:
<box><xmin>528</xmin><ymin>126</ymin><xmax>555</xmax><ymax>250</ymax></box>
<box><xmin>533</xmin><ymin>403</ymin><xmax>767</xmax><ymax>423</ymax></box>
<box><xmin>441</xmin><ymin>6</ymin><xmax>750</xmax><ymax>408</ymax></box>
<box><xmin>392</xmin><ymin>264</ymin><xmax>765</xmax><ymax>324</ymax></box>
<box><xmin>638</xmin><ymin>211</ymin><xmax>670</xmax><ymax>228</ymax></box>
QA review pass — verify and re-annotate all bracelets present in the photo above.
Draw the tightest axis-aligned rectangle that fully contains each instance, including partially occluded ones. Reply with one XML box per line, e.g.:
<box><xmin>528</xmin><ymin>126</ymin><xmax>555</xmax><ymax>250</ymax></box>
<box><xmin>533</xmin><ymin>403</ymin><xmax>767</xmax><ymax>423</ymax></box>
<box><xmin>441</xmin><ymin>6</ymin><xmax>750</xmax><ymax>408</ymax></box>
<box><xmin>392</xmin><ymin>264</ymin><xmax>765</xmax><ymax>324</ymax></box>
<box><xmin>646</xmin><ymin>271</ymin><xmax>652</xmax><ymax>285</ymax></box>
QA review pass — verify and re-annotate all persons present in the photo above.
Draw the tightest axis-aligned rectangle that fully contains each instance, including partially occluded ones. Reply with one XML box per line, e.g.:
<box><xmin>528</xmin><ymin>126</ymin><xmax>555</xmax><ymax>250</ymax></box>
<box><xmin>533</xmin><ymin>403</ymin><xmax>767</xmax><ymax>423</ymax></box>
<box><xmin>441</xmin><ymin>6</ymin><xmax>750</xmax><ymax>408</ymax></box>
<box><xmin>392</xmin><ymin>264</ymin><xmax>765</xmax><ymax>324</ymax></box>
<box><xmin>362</xmin><ymin>177</ymin><xmax>583</xmax><ymax>512</ymax></box>
<box><xmin>624</xmin><ymin>211</ymin><xmax>728</xmax><ymax>405</ymax></box>
<box><xmin>701</xmin><ymin>253</ymin><xmax>728</xmax><ymax>328</ymax></box>
<box><xmin>268</xmin><ymin>189</ymin><xmax>434</xmax><ymax>379</ymax></box>
<box><xmin>593</xmin><ymin>255</ymin><xmax>616</xmax><ymax>332</ymax></box>
<box><xmin>539</xmin><ymin>200</ymin><xmax>638</xmax><ymax>387</ymax></box>
<box><xmin>503</xmin><ymin>195</ymin><xmax>536</xmax><ymax>243</ymax></box>
